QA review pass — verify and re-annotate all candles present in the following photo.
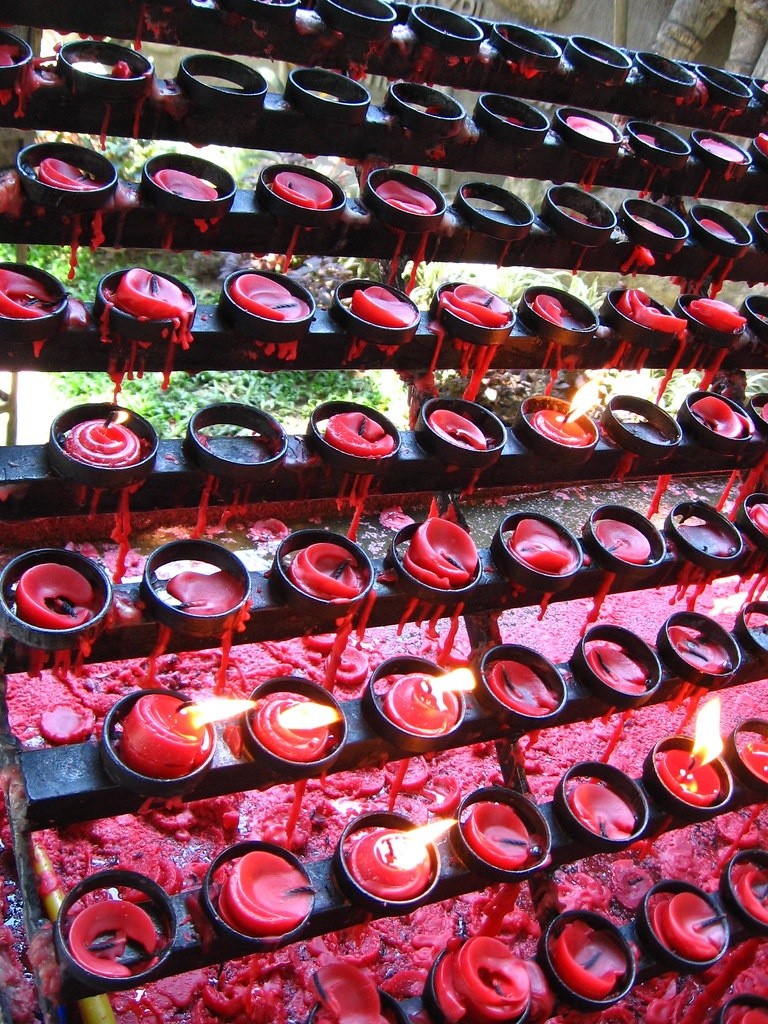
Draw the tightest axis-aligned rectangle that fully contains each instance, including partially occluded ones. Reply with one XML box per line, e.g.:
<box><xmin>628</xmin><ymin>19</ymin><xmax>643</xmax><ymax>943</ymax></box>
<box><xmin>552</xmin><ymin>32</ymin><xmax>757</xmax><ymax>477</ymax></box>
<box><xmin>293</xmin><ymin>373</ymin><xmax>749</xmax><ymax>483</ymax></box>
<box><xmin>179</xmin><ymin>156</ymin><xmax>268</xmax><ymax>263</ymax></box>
<box><xmin>532</xmin><ymin>377</ymin><xmax>603</xmax><ymax>444</ymax></box>
<box><xmin>165</xmin><ymin>566</ymin><xmax>244</xmax><ymax>616</ymax></box>
<box><xmin>289</xmin><ymin>543</ymin><xmax>368</xmax><ymax>600</ymax></box>
<box><xmin>670</xmin><ymin>625</ymin><xmax>728</xmax><ymax>675</ymax></box>
<box><xmin>589</xmin><ymin>643</ymin><xmax>647</xmax><ymax>693</ymax></box>
<box><xmin>298</xmin><ymin>956</ymin><xmax>382</xmax><ymax>1024</ymax></box>
<box><xmin>507</xmin><ymin>517</ymin><xmax>575</xmax><ymax>576</ymax></box>
<box><xmin>737</xmin><ymin>864</ymin><xmax>768</xmax><ymax>924</ymax></box>
<box><xmin>403</xmin><ymin>515</ymin><xmax>479</xmax><ymax>589</ymax></box>
<box><xmin>349</xmin><ymin>816</ymin><xmax>458</xmax><ymax>900</ymax></box>
<box><xmin>384</xmin><ymin>670</ymin><xmax>479</xmax><ymax>735</ymax></box>
<box><xmin>119</xmin><ymin>687</ymin><xmax>257</xmax><ymax>778</ymax></box>
<box><xmin>67</xmin><ymin>901</ymin><xmax>161</xmax><ymax>978</ymax></box>
<box><xmin>691</xmin><ymin>396</ymin><xmax>745</xmax><ymax>440</ymax></box>
<box><xmin>67</xmin><ymin>405</ymin><xmax>141</xmax><ymax>467</ymax></box>
<box><xmin>1</xmin><ymin>37</ymin><xmax>768</xmax><ymax>385</ymax></box>
<box><xmin>16</xmin><ymin>562</ymin><xmax>95</xmax><ymax>631</ymax></box>
<box><xmin>219</xmin><ymin>849</ymin><xmax>316</xmax><ymax>938</ymax></box>
<box><xmin>324</xmin><ymin>409</ymin><xmax>395</xmax><ymax>460</ymax></box>
<box><xmin>252</xmin><ymin>690</ymin><xmax>342</xmax><ymax>759</ymax></box>
<box><xmin>656</xmin><ymin>890</ymin><xmax>729</xmax><ymax>959</ymax></box>
<box><xmin>556</xmin><ymin>921</ymin><xmax>628</xmax><ymax>995</ymax></box>
<box><xmin>750</xmin><ymin>502</ymin><xmax>768</xmax><ymax>533</ymax></box>
<box><xmin>435</xmin><ymin>935</ymin><xmax>532</xmax><ymax>1023</ymax></box>
<box><xmin>491</xmin><ymin>660</ymin><xmax>557</xmax><ymax>717</ymax></box>
<box><xmin>595</xmin><ymin>517</ymin><xmax>651</xmax><ymax>565</ymax></box>
<box><xmin>743</xmin><ymin>743</ymin><xmax>768</xmax><ymax>777</ymax></box>
<box><xmin>570</xmin><ymin>785</ymin><xmax>635</xmax><ymax>842</ymax></box>
<box><xmin>659</xmin><ymin>694</ymin><xmax>726</xmax><ymax>805</ymax></box>
<box><xmin>464</xmin><ymin>800</ymin><xmax>533</xmax><ymax>869</ymax></box>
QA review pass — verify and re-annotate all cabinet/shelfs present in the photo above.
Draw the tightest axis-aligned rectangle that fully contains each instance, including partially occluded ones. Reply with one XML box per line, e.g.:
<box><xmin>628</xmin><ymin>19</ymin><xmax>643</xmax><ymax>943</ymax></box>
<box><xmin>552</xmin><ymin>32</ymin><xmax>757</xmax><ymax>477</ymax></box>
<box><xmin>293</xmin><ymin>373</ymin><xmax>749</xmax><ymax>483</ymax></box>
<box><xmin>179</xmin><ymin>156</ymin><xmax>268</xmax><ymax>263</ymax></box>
<box><xmin>0</xmin><ymin>1</ymin><xmax>768</xmax><ymax>1024</ymax></box>
